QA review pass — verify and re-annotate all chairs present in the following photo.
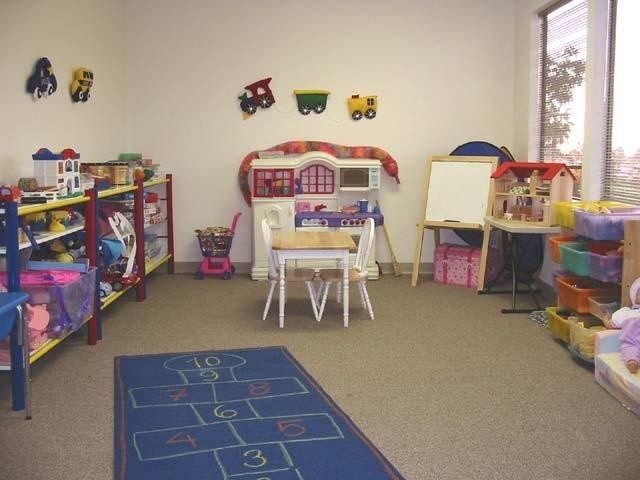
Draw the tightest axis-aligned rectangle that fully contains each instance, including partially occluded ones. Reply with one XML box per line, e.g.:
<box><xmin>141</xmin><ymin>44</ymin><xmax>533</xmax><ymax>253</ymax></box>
<box><xmin>316</xmin><ymin>218</ymin><xmax>375</xmax><ymax>321</ymax></box>
<box><xmin>262</xmin><ymin>218</ymin><xmax>320</xmax><ymax>321</ymax></box>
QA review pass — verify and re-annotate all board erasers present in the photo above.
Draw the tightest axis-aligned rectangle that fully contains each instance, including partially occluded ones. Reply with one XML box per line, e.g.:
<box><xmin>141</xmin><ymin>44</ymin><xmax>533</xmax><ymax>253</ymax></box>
<box><xmin>443</xmin><ymin>219</ymin><xmax>461</xmax><ymax>223</ymax></box>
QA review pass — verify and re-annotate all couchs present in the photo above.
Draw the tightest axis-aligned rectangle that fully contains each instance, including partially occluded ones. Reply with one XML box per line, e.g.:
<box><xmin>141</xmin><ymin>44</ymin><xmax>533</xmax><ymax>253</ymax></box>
<box><xmin>594</xmin><ymin>329</ymin><xmax>639</xmax><ymax>419</ymax></box>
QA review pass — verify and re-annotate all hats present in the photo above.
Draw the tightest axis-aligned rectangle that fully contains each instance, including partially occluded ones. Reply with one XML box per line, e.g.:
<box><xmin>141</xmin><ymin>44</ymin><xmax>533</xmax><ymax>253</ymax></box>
<box><xmin>630</xmin><ymin>277</ymin><xmax>640</xmax><ymax>309</ymax></box>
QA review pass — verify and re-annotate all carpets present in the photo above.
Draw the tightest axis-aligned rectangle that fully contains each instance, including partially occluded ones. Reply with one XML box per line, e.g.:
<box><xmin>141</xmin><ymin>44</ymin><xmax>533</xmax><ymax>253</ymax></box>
<box><xmin>113</xmin><ymin>345</ymin><xmax>403</xmax><ymax>480</ymax></box>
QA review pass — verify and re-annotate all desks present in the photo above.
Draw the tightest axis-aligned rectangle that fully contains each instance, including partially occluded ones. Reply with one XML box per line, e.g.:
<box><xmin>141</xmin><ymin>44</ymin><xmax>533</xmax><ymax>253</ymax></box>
<box><xmin>272</xmin><ymin>232</ymin><xmax>356</xmax><ymax>328</ymax></box>
<box><xmin>477</xmin><ymin>216</ymin><xmax>561</xmax><ymax>313</ymax></box>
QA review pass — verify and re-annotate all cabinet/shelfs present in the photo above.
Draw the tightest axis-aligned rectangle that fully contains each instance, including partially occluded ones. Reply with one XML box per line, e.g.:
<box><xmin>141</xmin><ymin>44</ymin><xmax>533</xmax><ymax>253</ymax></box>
<box><xmin>2</xmin><ymin>190</ymin><xmax>97</xmax><ymax>411</ymax></box>
<box><xmin>136</xmin><ymin>174</ymin><xmax>174</xmax><ymax>301</ymax></box>
<box><xmin>89</xmin><ymin>180</ymin><xmax>144</xmax><ymax>340</ymax></box>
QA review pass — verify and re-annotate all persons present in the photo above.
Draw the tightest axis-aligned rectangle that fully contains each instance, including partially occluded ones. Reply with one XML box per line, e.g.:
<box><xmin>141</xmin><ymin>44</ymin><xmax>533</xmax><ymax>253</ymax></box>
<box><xmin>620</xmin><ymin>277</ymin><xmax>640</xmax><ymax>374</ymax></box>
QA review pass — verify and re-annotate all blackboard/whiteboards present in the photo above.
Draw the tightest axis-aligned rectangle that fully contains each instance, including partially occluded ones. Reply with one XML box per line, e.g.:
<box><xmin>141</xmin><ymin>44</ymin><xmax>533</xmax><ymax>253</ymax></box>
<box><xmin>411</xmin><ymin>155</ymin><xmax>499</xmax><ymax>294</ymax></box>
